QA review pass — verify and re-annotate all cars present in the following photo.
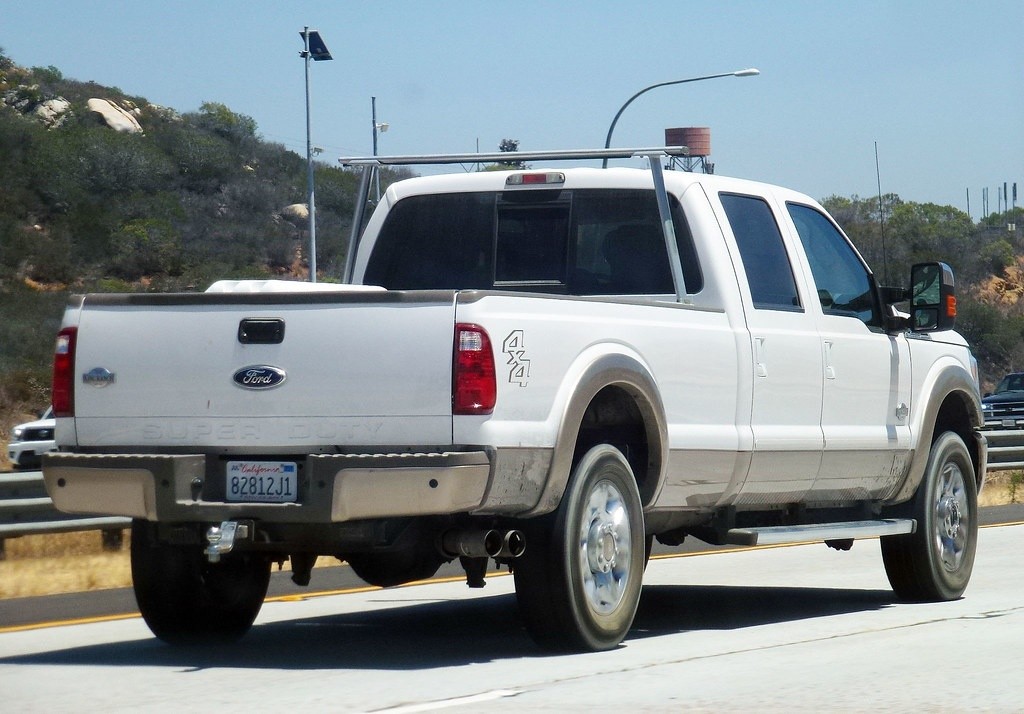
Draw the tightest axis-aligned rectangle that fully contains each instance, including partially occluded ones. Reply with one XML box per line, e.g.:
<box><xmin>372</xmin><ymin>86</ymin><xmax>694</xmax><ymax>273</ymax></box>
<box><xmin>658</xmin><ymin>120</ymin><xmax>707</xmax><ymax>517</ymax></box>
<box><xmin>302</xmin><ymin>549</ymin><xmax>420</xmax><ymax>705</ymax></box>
<box><xmin>982</xmin><ymin>373</ymin><xmax>1024</xmax><ymax>429</ymax></box>
<box><xmin>5</xmin><ymin>406</ymin><xmax>58</xmax><ymax>471</ymax></box>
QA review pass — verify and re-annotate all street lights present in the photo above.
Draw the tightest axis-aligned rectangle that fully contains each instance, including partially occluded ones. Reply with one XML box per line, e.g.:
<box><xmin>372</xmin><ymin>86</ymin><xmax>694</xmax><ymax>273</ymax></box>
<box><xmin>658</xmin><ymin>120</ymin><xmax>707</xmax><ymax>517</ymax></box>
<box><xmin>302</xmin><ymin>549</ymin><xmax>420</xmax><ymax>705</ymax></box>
<box><xmin>372</xmin><ymin>123</ymin><xmax>389</xmax><ymax>199</ymax></box>
<box><xmin>600</xmin><ymin>67</ymin><xmax>761</xmax><ymax>168</ymax></box>
<box><xmin>301</xmin><ymin>29</ymin><xmax>331</xmax><ymax>281</ymax></box>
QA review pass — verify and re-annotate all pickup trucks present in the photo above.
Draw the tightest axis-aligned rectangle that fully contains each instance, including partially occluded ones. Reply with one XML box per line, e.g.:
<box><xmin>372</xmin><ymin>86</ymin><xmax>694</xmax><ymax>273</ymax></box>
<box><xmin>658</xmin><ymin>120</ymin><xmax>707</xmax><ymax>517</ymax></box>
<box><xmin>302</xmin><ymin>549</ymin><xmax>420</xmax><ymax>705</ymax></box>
<box><xmin>41</xmin><ymin>142</ymin><xmax>988</xmax><ymax>650</ymax></box>
